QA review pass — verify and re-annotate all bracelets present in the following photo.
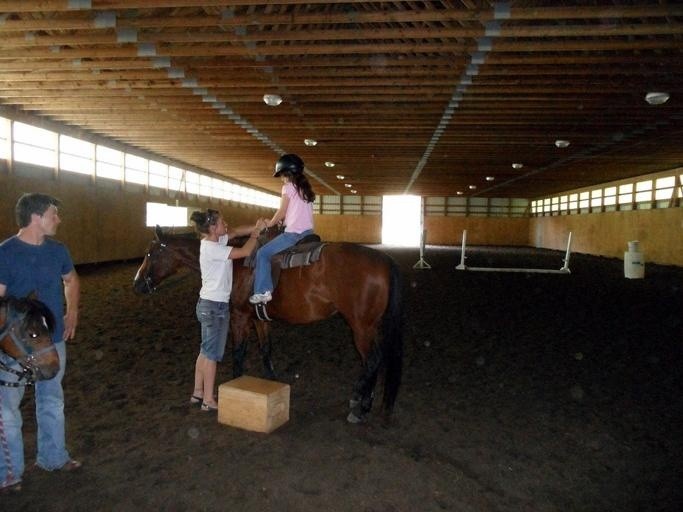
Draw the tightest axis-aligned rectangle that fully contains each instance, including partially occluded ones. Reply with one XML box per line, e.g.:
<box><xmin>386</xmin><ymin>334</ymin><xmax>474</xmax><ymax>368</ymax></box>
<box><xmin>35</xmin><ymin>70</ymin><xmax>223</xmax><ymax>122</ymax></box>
<box><xmin>248</xmin><ymin>227</ymin><xmax>260</xmax><ymax>239</ymax></box>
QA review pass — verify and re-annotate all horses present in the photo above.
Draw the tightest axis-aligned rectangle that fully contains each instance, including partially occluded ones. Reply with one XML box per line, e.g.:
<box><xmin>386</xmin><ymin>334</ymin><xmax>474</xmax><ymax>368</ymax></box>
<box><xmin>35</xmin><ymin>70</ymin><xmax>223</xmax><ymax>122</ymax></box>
<box><xmin>0</xmin><ymin>287</ymin><xmax>60</xmax><ymax>381</ymax></box>
<box><xmin>132</xmin><ymin>225</ymin><xmax>404</xmax><ymax>426</ymax></box>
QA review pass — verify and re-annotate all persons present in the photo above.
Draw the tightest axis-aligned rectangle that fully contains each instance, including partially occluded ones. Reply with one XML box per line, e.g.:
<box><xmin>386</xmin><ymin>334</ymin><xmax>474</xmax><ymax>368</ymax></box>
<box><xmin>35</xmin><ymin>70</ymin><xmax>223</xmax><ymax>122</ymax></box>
<box><xmin>0</xmin><ymin>190</ymin><xmax>82</xmax><ymax>493</ymax></box>
<box><xmin>243</xmin><ymin>153</ymin><xmax>315</xmax><ymax>304</ymax></box>
<box><xmin>178</xmin><ymin>206</ymin><xmax>268</xmax><ymax>413</ymax></box>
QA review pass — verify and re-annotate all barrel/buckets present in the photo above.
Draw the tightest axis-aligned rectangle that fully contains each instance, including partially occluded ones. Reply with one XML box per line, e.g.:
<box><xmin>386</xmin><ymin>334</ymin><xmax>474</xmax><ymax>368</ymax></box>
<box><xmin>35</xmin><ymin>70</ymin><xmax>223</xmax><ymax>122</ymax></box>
<box><xmin>628</xmin><ymin>241</ymin><xmax>640</xmax><ymax>251</ymax></box>
<box><xmin>624</xmin><ymin>251</ymin><xmax>645</xmax><ymax>279</ymax></box>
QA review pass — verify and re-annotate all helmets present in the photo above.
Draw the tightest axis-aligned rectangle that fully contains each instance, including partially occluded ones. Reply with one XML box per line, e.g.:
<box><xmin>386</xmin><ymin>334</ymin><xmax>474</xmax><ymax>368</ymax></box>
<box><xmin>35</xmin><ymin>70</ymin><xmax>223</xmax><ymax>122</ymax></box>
<box><xmin>273</xmin><ymin>153</ymin><xmax>305</xmax><ymax>178</ymax></box>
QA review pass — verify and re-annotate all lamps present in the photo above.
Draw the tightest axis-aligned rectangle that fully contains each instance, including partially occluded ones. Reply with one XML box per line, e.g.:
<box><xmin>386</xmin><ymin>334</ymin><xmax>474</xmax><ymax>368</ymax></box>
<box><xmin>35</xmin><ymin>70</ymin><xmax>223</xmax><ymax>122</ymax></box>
<box><xmin>262</xmin><ymin>92</ymin><xmax>357</xmax><ymax>197</ymax></box>
<box><xmin>455</xmin><ymin>91</ymin><xmax>672</xmax><ymax>197</ymax></box>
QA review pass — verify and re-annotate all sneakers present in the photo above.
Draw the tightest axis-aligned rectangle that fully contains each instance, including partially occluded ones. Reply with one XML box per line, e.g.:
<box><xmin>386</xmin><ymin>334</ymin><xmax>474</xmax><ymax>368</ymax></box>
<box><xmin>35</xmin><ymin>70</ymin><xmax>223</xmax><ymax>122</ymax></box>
<box><xmin>0</xmin><ymin>482</ymin><xmax>25</xmax><ymax>497</ymax></box>
<box><xmin>249</xmin><ymin>290</ymin><xmax>272</xmax><ymax>304</ymax></box>
<box><xmin>34</xmin><ymin>459</ymin><xmax>82</xmax><ymax>472</ymax></box>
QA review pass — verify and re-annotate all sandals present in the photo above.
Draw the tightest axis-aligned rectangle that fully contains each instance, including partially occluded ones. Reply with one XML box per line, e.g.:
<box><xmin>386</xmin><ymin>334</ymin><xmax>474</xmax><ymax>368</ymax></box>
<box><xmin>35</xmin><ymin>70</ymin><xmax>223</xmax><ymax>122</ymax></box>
<box><xmin>190</xmin><ymin>394</ymin><xmax>218</xmax><ymax>412</ymax></box>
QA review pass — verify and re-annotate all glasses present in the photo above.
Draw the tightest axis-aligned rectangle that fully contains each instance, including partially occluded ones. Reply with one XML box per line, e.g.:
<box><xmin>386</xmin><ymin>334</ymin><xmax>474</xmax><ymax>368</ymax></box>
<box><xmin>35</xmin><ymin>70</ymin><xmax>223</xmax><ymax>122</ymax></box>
<box><xmin>205</xmin><ymin>208</ymin><xmax>216</xmax><ymax>225</ymax></box>
